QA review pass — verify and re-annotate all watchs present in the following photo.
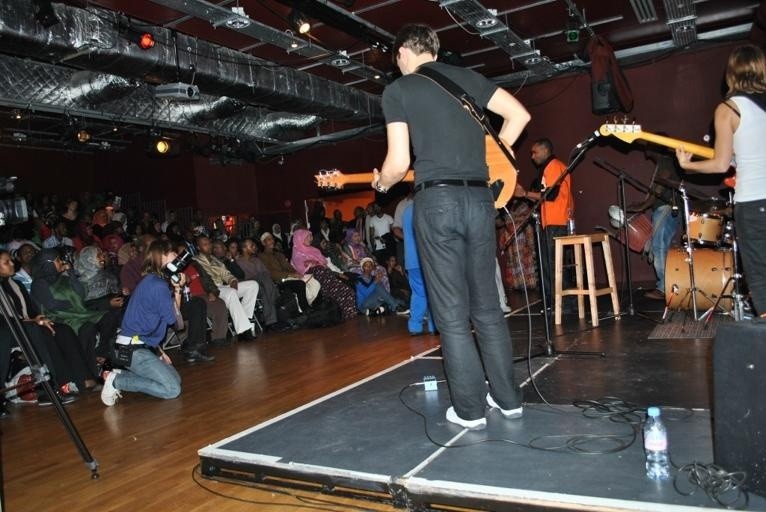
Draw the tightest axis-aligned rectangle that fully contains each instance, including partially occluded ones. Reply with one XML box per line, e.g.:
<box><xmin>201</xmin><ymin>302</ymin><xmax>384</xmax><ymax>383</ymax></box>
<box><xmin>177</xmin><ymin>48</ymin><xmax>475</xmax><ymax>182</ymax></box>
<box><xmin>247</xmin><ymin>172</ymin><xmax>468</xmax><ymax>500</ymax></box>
<box><xmin>375</xmin><ymin>181</ymin><xmax>387</xmax><ymax>194</ymax></box>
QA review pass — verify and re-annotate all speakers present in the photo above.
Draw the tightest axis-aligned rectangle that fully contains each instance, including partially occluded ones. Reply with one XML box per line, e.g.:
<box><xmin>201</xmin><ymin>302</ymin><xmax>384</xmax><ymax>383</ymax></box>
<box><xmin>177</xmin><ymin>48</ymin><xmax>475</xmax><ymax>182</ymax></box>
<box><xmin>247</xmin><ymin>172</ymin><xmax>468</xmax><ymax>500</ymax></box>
<box><xmin>712</xmin><ymin>319</ymin><xmax>765</xmax><ymax>495</ymax></box>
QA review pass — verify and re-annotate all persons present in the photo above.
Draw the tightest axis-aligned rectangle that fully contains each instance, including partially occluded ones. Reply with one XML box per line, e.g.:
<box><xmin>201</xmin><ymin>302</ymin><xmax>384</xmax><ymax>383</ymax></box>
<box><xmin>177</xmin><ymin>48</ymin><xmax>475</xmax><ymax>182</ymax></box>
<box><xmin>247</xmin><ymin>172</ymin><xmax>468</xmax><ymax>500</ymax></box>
<box><xmin>192</xmin><ymin>234</ymin><xmax>260</xmax><ymax>342</ymax></box>
<box><xmin>0</xmin><ymin>322</ymin><xmax>78</xmax><ymax>420</ymax></box>
<box><xmin>42</xmin><ymin>220</ymin><xmax>74</xmax><ymax>278</ymax></box>
<box><xmin>38</xmin><ymin>194</ymin><xmax>56</xmax><ymax>223</ymax></box>
<box><xmin>13</xmin><ymin>244</ymin><xmax>40</xmax><ymax>294</ymax></box>
<box><xmin>156</xmin><ymin>231</ymin><xmax>171</xmax><ymax>240</ymax></box>
<box><xmin>349</xmin><ymin>206</ymin><xmax>366</xmax><ymax>241</ymax></box>
<box><xmin>513</xmin><ymin>138</ymin><xmax>579</xmax><ymax>315</ymax></box>
<box><xmin>86</xmin><ymin>208</ymin><xmax>129</xmax><ymax>245</ymax></box>
<box><xmin>225</xmin><ymin>238</ymin><xmax>238</xmax><ymax>257</ymax></box>
<box><xmin>236</xmin><ymin>238</ymin><xmax>301</xmax><ymax>335</ymax></box>
<box><xmin>172</xmin><ymin>240</ymin><xmax>232</xmax><ymax>348</ymax></box>
<box><xmin>0</xmin><ymin>248</ymin><xmax>102</xmax><ymax>393</ymax></box>
<box><xmin>674</xmin><ymin>44</ymin><xmax>766</xmax><ymax>318</ymax></box>
<box><xmin>355</xmin><ymin>256</ymin><xmax>410</xmax><ymax>317</ymax></box>
<box><xmin>255</xmin><ymin>220</ymin><xmax>264</xmax><ymax>238</ymax></box>
<box><xmin>71</xmin><ymin>246</ymin><xmax>130</xmax><ymax>325</ymax></box>
<box><xmin>120</xmin><ymin>234</ymin><xmax>216</xmax><ymax>363</ymax></box>
<box><xmin>60</xmin><ymin>198</ymin><xmax>81</xmax><ymax>225</ymax></box>
<box><xmin>369</xmin><ymin>200</ymin><xmax>393</xmax><ymax>267</ymax></box>
<box><xmin>290</xmin><ymin>229</ymin><xmax>356</xmax><ymax>319</ymax></box>
<box><xmin>315</xmin><ymin>237</ymin><xmax>360</xmax><ymax>288</ymax></box>
<box><xmin>8</xmin><ymin>223</ymin><xmax>42</xmax><ymax>262</ymax></box>
<box><xmin>26</xmin><ymin>192</ymin><xmax>35</xmax><ymax>219</ymax></box>
<box><xmin>209</xmin><ymin>219</ymin><xmax>229</xmax><ymax>238</ymax></box>
<box><xmin>626</xmin><ymin>131</ymin><xmax>680</xmax><ymax>300</ymax></box>
<box><xmin>101</xmin><ymin>248</ymin><xmax>120</xmax><ymax>273</ymax></box>
<box><xmin>332</xmin><ymin>209</ymin><xmax>348</xmax><ymax>240</ymax></box>
<box><xmin>212</xmin><ymin>239</ymin><xmax>245</xmax><ymax>281</ymax></box>
<box><xmin>178</xmin><ymin>229</ymin><xmax>197</xmax><ymax>255</ymax></box>
<box><xmin>317</xmin><ymin>217</ymin><xmax>337</xmax><ymax>243</ymax></box>
<box><xmin>272</xmin><ymin>224</ymin><xmax>288</xmax><ymax>253</ymax></box>
<box><xmin>257</xmin><ymin>232</ymin><xmax>322</xmax><ymax>312</ymax></box>
<box><xmin>117</xmin><ymin>238</ymin><xmax>151</xmax><ymax>287</ymax></box>
<box><xmin>275</xmin><ymin>239</ymin><xmax>281</xmax><ymax>252</ymax></box>
<box><xmin>30</xmin><ymin>248</ymin><xmax>117</xmax><ymax>386</ymax></box>
<box><xmin>100</xmin><ymin>237</ymin><xmax>186</xmax><ymax>407</ymax></box>
<box><xmin>103</xmin><ymin>233</ymin><xmax>122</xmax><ymax>265</ymax></box>
<box><xmin>495</xmin><ymin>257</ymin><xmax>512</xmax><ymax>312</ymax></box>
<box><xmin>371</xmin><ymin>22</ymin><xmax>531</xmax><ymax>432</ymax></box>
<box><xmin>392</xmin><ymin>182</ymin><xmax>414</xmax><ymax>239</ymax></box>
<box><xmin>365</xmin><ymin>202</ymin><xmax>375</xmax><ymax>253</ymax></box>
<box><xmin>386</xmin><ymin>256</ymin><xmax>409</xmax><ymax>298</ymax></box>
<box><xmin>401</xmin><ymin>202</ymin><xmax>437</xmax><ymax>335</ymax></box>
<box><xmin>161</xmin><ymin>209</ymin><xmax>176</xmax><ymax>232</ymax></box>
<box><xmin>51</xmin><ymin>193</ymin><xmax>60</xmax><ymax>212</ymax></box>
<box><xmin>344</xmin><ymin>228</ymin><xmax>390</xmax><ymax>293</ymax></box>
<box><xmin>326</xmin><ymin>241</ymin><xmax>352</xmax><ymax>270</ymax></box>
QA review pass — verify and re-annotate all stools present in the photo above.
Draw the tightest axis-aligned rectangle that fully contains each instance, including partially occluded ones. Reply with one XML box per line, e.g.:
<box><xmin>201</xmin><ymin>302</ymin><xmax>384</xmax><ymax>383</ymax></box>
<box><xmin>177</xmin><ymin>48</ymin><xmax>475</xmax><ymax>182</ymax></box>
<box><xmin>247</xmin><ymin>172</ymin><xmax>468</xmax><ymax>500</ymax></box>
<box><xmin>552</xmin><ymin>231</ymin><xmax>623</xmax><ymax>326</ymax></box>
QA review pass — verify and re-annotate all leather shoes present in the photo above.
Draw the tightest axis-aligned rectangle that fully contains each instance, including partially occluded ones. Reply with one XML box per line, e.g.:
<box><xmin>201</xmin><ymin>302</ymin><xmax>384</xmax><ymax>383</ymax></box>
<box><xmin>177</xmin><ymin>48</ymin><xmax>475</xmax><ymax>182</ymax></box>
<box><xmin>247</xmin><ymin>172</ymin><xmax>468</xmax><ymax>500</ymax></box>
<box><xmin>643</xmin><ymin>289</ymin><xmax>665</xmax><ymax>300</ymax></box>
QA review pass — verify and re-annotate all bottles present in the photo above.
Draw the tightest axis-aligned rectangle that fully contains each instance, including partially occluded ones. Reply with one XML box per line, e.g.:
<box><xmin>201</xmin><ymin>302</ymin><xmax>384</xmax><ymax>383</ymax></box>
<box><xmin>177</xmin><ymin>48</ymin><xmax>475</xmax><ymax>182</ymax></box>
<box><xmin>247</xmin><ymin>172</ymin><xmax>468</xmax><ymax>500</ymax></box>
<box><xmin>567</xmin><ymin>209</ymin><xmax>576</xmax><ymax>236</ymax></box>
<box><xmin>644</xmin><ymin>408</ymin><xmax>670</xmax><ymax>480</ymax></box>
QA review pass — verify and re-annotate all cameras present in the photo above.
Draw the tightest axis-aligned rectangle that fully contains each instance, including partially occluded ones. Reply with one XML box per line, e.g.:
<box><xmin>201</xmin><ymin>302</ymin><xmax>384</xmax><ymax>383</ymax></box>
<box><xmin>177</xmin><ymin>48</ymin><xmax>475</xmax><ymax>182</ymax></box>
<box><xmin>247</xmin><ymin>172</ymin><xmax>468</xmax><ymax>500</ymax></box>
<box><xmin>160</xmin><ymin>248</ymin><xmax>192</xmax><ymax>284</ymax></box>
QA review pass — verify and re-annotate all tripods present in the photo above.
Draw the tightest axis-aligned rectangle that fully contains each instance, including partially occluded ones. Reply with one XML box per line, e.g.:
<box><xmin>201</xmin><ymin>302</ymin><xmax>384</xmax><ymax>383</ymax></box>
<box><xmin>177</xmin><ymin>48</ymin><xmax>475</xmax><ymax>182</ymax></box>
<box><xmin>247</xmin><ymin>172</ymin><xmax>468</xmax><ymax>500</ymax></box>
<box><xmin>587</xmin><ymin>157</ymin><xmax>664</xmax><ymax>324</ymax></box>
<box><xmin>503</xmin><ymin>148</ymin><xmax>605</xmax><ymax>364</ymax></box>
<box><xmin>1</xmin><ymin>174</ymin><xmax>99</xmax><ymax>512</ymax></box>
<box><xmin>705</xmin><ymin>191</ymin><xmax>763</xmax><ymax>331</ymax></box>
<box><xmin>666</xmin><ymin>182</ymin><xmax>735</xmax><ymax>332</ymax></box>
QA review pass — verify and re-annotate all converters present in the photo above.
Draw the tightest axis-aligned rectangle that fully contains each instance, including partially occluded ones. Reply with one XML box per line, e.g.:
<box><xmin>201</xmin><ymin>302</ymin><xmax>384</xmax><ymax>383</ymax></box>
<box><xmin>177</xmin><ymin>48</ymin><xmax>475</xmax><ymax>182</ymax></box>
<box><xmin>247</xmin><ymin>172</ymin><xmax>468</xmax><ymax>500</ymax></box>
<box><xmin>424</xmin><ymin>374</ymin><xmax>438</xmax><ymax>390</ymax></box>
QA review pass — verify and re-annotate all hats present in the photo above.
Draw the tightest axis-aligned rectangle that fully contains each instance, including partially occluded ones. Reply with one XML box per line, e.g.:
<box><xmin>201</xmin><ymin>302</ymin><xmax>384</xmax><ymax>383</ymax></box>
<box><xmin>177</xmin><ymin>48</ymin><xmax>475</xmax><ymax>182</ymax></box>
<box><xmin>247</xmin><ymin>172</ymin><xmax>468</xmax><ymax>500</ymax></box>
<box><xmin>360</xmin><ymin>256</ymin><xmax>374</xmax><ymax>271</ymax></box>
<box><xmin>260</xmin><ymin>232</ymin><xmax>270</xmax><ymax>242</ymax></box>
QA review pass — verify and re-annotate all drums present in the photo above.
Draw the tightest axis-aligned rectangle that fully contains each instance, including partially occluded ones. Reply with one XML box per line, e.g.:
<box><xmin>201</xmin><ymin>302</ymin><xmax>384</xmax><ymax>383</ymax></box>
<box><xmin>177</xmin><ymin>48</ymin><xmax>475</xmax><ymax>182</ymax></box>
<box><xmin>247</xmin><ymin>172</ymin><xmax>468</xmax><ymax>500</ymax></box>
<box><xmin>614</xmin><ymin>212</ymin><xmax>654</xmax><ymax>253</ymax></box>
<box><xmin>664</xmin><ymin>248</ymin><xmax>734</xmax><ymax>312</ymax></box>
<box><xmin>688</xmin><ymin>212</ymin><xmax>726</xmax><ymax>246</ymax></box>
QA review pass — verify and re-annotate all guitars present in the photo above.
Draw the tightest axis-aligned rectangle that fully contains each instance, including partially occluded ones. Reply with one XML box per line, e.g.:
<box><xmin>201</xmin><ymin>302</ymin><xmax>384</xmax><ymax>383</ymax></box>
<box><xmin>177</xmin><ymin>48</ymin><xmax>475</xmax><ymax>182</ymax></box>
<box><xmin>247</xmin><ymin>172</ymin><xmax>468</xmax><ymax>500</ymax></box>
<box><xmin>600</xmin><ymin>116</ymin><xmax>736</xmax><ymax>188</ymax></box>
<box><xmin>314</xmin><ymin>133</ymin><xmax>518</xmax><ymax>210</ymax></box>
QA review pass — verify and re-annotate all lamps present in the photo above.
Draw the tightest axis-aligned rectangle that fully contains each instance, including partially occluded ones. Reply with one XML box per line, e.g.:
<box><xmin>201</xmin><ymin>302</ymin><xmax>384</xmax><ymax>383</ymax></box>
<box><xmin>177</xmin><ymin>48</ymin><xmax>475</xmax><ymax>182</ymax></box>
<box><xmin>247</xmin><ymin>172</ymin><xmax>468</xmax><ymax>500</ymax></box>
<box><xmin>116</xmin><ymin>24</ymin><xmax>157</xmax><ymax>50</ymax></box>
<box><xmin>524</xmin><ymin>55</ymin><xmax>543</xmax><ymax>67</ymax></box>
<box><xmin>70</xmin><ymin>126</ymin><xmax>93</xmax><ymax>145</ymax></box>
<box><xmin>562</xmin><ymin>17</ymin><xmax>581</xmax><ymax>44</ymax></box>
<box><xmin>140</xmin><ymin>131</ymin><xmax>171</xmax><ymax>155</ymax></box>
<box><xmin>475</xmin><ymin>17</ymin><xmax>500</xmax><ymax>31</ymax></box>
<box><xmin>287</xmin><ymin>9</ymin><xmax>311</xmax><ymax>35</ymax></box>
<box><xmin>224</xmin><ymin>17</ymin><xmax>252</xmax><ymax>29</ymax></box>
<box><xmin>675</xmin><ymin>23</ymin><xmax>694</xmax><ymax>34</ymax></box>
<box><xmin>329</xmin><ymin>53</ymin><xmax>353</xmax><ymax>70</ymax></box>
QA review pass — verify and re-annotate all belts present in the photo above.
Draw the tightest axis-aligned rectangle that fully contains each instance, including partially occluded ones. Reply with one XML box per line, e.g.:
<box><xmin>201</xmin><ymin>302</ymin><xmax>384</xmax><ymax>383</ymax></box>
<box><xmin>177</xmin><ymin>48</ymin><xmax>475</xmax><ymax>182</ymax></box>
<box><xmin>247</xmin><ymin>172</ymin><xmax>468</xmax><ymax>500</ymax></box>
<box><xmin>112</xmin><ymin>342</ymin><xmax>148</xmax><ymax>351</ymax></box>
<box><xmin>413</xmin><ymin>179</ymin><xmax>491</xmax><ymax>194</ymax></box>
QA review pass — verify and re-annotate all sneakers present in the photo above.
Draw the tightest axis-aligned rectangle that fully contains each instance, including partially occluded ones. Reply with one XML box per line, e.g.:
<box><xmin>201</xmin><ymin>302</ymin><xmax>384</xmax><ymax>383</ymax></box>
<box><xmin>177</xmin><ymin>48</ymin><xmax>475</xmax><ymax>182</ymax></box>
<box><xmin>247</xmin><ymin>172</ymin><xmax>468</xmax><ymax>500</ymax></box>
<box><xmin>407</xmin><ymin>330</ymin><xmax>422</xmax><ymax>336</ymax></box>
<box><xmin>487</xmin><ymin>392</ymin><xmax>524</xmax><ymax>420</ymax></box>
<box><xmin>100</xmin><ymin>372</ymin><xmax>123</xmax><ymax>407</ymax></box>
<box><xmin>366</xmin><ymin>308</ymin><xmax>376</xmax><ymax>317</ymax></box>
<box><xmin>237</xmin><ymin>331</ymin><xmax>259</xmax><ymax>343</ymax></box>
<box><xmin>0</xmin><ymin>404</ymin><xmax>10</xmax><ymax>419</ymax></box>
<box><xmin>185</xmin><ymin>350</ymin><xmax>216</xmax><ymax>363</ymax></box>
<box><xmin>209</xmin><ymin>338</ymin><xmax>231</xmax><ymax>349</ymax></box>
<box><xmin>395</xmin><ymin>306</ymin><xmax>410</xmax><ymax>314</ymax></box>
<box><xmin>426</xmin><ymin>330</ymin><xmax>440</xmax><ymax>336</ymax></box>
<box><xmin>112</xmin><ymin>369</ymin><xmax>122</xmax><ymax>374</ymax></box>
<box><xmin>274</xmin><ymin>322</ymin><xmax>292</xmax><ymax>331</ymax></box>
<box><xmin>37</xmin><ymin>391</ymin><xmax>76</xmax><ymax>406</ymax></box>
<box><xmin>445</xmin><ymin>407</ymin><xmax>487</xmax><ymax>431</ymax></box>
<box><xmin>266</xmin><ymin>325</ymin><xmax>288</xmax><ymax>336</ymax></box>
<box><xmin>750</xmin><ymin>314</ymin><xmax>766</xmax><ymax>323</ymax></box>
<box><xmin>499</xmin><ymin>303</ymin><xmax>513</xmax><ymax>313</ymax></box>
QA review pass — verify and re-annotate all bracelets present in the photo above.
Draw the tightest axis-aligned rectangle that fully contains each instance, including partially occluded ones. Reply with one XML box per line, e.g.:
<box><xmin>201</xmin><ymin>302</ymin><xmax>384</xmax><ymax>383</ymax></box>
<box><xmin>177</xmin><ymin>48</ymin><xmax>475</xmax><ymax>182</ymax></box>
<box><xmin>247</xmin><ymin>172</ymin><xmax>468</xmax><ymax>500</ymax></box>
<box><xmin>173</xmin><ymin>289</ymin><xmax>183</xmax><ymax>294</ymax></box>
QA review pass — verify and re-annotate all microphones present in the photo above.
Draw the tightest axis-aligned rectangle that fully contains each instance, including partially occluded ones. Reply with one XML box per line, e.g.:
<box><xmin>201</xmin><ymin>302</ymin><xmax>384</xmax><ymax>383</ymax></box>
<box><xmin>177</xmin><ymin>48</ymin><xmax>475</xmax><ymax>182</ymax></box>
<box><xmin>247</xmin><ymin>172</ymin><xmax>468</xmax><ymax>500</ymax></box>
<box><xmin>574</xmin><ymin>128</ymin><xmax>601</xmax><ymax>149</ymax></box>
<box><xmin>671</xmin><ymin>187</ymin><xmax>679</xmax><ymax>217</ymax></box>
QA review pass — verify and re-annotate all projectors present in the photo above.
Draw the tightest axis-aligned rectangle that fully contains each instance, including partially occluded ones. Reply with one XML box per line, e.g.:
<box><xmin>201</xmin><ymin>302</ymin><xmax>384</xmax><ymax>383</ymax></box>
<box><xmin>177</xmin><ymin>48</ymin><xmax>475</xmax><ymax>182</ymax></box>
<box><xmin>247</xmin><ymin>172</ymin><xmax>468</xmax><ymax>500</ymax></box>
<box><xmin>154</xmin><ymin>82</ymin><xmax>200</xmax><ymax>101</ymax></box>
